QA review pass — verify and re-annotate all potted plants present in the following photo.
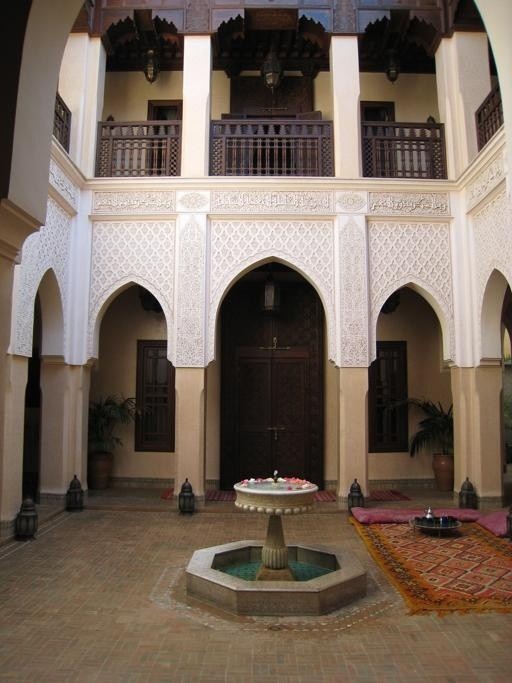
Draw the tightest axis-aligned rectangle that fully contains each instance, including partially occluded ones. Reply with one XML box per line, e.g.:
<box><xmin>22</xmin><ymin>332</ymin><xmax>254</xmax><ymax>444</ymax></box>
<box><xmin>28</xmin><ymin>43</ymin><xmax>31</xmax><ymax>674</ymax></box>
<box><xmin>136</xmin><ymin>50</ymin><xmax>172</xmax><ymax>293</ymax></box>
<box><xmin>399</xmin><ymin>398</ymin><xmax>454</xmax><ymax>492</ymax></box>
<box><xmin>89</xmin><ymin>393</ymin><xmax>136</xmax><ymax>490</ymax></box>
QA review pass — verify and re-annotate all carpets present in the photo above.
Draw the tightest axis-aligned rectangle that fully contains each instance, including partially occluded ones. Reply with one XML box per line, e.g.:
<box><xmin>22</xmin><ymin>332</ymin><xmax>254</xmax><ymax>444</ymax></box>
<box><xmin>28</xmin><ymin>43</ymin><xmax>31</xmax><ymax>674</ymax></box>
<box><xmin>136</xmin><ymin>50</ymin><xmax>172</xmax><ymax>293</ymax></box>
<box><xmin>346</xmin><ymin>503</ymin><xmax>512</xmax><ymax>615</ymax></box>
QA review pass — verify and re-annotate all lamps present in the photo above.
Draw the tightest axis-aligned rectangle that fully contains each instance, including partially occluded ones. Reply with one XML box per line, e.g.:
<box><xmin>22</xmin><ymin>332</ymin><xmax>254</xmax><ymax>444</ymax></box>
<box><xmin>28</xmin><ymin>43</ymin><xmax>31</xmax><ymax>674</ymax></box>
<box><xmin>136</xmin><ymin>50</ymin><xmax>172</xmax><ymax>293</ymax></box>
<box><xmin>348</xmin><ymin>478</ymin><xmax>363</xmax><ymax>503</ymax></box>
<box><xmin>263</xmin><ymin>277</ymin><xmax>280</xmax><ymax>313</ymax></box>
<box><xmin>64</xmin><ymin>476</ymin><xmax>84</xmax><ymax>513</ymax></box>
<box><xmin>458</xmin><ymin>476</ymin><xmax>479</xmax><ymax>509</ymax></box>
<box><xmin>178</xmin><ymin>478</ymin><xmax>195</xmax><ymax>515</ymax></box>
<box><xmin>143</xmin><ymin>48</ymin><xmax>399</xmax><ymax>94</ymax></box>
<box><xmin>15</xmin><ymin>497</ymin><xmax>39</xmax><ymax>541</ymax></box>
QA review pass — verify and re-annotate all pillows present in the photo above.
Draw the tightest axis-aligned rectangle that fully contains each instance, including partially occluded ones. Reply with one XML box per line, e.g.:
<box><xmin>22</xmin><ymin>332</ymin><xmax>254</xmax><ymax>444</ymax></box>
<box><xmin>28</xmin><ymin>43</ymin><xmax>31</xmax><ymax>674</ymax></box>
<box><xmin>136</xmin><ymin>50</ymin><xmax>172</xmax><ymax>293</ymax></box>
<box><xmin>349</xmin><ymin>506</ymin><xmax>512</xmax><ymax>536</ymax></box>
